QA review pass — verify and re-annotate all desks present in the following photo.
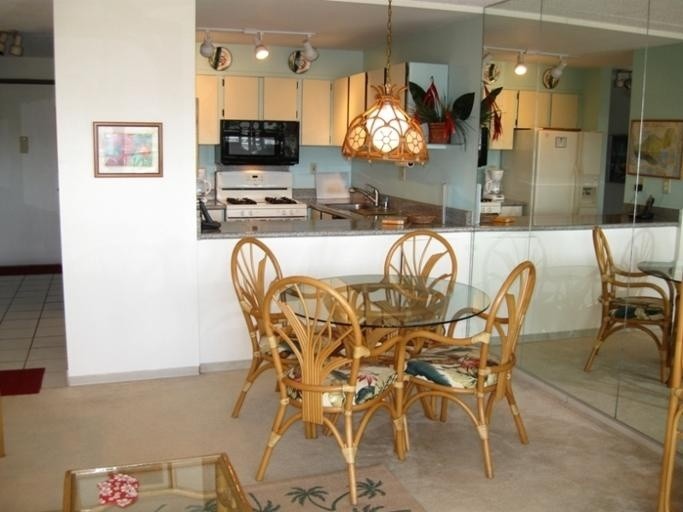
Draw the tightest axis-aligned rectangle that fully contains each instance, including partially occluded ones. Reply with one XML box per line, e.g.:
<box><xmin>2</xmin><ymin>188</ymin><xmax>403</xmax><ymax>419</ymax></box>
<box><xmin>281</xmin><ymin>274</ymin><xmax>490</xmax><ymax>452</ymax></box>
<box><xmin>636</xmin><ymin>258</ymin><xmax>683</xmax><ymax>386</ymax></box>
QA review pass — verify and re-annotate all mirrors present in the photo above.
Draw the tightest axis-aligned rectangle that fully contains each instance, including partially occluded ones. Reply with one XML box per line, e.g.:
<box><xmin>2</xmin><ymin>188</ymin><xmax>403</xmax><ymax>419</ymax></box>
<box><xmin>627</xmin><ymin>119</ymin><xmax>683</xmax><ymax>179</ymax></box>
<box><xmin>467</xmin><ymin>0</ymin><xmax>683</xmax><ymax>459</ymax></box>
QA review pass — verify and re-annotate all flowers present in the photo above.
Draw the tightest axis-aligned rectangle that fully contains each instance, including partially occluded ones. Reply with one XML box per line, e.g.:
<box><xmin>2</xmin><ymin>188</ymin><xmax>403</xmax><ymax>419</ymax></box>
<box><xmin>480</xmin><ymin>81</ymin><xmax>505</xmax><ymax>141</ymax></box>
<box><xmin>404</xmin><ymin>77</ymin><xmax>475</xmax><ymax>150</ymax></box>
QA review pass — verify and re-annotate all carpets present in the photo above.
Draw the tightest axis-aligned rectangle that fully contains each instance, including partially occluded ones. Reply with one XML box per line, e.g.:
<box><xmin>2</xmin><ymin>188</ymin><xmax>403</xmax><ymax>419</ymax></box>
<box><xmin>242</xmin><ymin>464</ymin><xmax>424</xmax><ymax>512</ymax></box>
<box><xmin>0</xmin><ymin>368</ymin><xmax>45</xmax><ymax>395</ymax></box>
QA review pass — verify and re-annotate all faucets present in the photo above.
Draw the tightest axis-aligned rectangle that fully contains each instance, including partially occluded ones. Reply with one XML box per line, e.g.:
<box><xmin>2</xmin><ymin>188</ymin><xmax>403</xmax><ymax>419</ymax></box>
<box><xmin>348</xmin><ymin>182</ymin><xmax>380</xmax><ymax>207</ymax></box>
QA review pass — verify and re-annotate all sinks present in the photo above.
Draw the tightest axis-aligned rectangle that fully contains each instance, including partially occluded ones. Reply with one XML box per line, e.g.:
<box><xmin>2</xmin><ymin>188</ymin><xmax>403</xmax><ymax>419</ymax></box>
<box><xmin>326</xmin><ymin>204</ymin><xmax>375</xmax><ymax>209</ymax></box>
<box><xmin>351</xmin><ymin>209</ymin><xmax>402</xmax><ymax>216</ymax></box>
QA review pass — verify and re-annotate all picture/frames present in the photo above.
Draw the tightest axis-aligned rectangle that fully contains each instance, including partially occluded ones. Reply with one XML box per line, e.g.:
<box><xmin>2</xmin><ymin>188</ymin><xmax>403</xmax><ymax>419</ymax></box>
<box><xmin>93</xmin><ymin>123</ymin><xmax>164</xmax><ymax>177</ymax></box>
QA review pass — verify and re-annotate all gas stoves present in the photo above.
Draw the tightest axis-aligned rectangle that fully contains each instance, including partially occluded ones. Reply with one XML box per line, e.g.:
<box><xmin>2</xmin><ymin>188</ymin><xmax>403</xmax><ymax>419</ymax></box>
<box><xmin>218</xmin><ymin>196</ymin><xmax>306</xmax><ymax>206</ymax></box>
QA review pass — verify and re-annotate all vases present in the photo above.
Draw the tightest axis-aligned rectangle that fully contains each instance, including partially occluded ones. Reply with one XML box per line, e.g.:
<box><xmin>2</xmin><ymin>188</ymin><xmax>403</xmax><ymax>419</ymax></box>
<box><xmin>429</xmin><ymin>124</ymin><xmax>450</xmax><ymax>145</ymax></box>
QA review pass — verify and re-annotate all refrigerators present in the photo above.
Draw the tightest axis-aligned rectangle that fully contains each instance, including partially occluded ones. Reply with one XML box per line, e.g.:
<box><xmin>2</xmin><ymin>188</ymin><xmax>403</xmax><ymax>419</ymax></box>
<box><xmin>529</xmin><ymin>215</ymin><xmax>603</xmax><ymax>228</ymax></box>
<box><xmin>501</xmin><ymin>127</ymin><xmax>607</xmax><ymax>216</ymax></box>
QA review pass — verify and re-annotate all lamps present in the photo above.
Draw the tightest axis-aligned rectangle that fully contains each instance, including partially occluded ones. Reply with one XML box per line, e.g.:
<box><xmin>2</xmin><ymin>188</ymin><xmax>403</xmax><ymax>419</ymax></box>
<box><xmin>200</xmin><ymin>31</ymin><xmax>319</xmax><ymax>62</ymax></box>
<box><xmin>342</xmin><ymin>0</ymin><xmax>428</xmax><ymax>167</ymax></box>
<box><xmin>483</xmin><ymin>50</ymin><xmax>568</xmax><ymax>78</ymax></box>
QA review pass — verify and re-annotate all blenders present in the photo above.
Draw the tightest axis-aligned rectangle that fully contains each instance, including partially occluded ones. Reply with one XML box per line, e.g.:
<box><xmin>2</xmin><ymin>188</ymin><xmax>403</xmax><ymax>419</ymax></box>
<box><xmin>486</xmin><ymin>169</ymin><xmax>505</xmax><ymax>199</ymax></box>
<box><xmin>196</xmin><ymin>175</ymin><xmax>212</xmax><ymax>202</ymax></box>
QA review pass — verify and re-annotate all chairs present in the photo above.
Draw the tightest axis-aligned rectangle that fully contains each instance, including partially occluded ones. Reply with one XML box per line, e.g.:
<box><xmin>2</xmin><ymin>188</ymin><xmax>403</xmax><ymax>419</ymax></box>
<box><xmin>394</xmin><ymin>261</ymin><xmax>535</xmax><ymax>479</ymax></box>
<box><xmin>230</xmin><ymin>229</ymin><xmax>458</xmax><ymax>418</ymax></box>
<box><xmin>583</xmin><ymin>228</ymin><xmax>673</xmax><ymax>383</ymax></box>
<box><xmin>256</xmin><ymin>277</ymin><xmax>409</xmax><ymax>503</ymax></box>
